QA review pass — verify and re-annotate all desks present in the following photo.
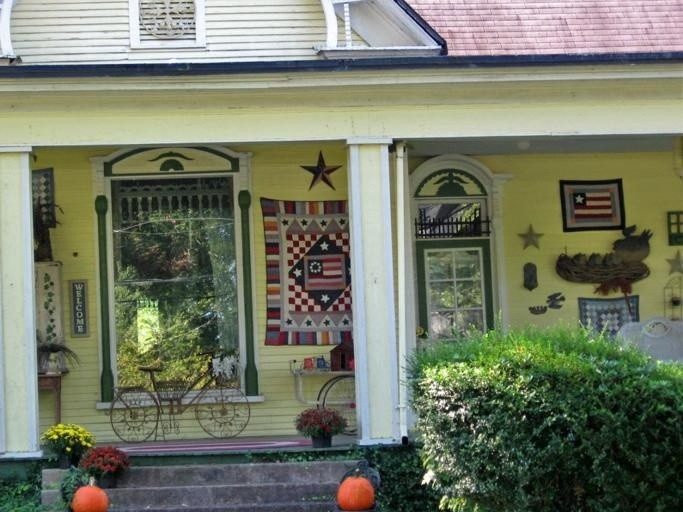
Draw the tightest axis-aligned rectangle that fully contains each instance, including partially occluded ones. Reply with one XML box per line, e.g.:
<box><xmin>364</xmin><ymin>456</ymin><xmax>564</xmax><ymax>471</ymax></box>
<box><xmin>37</xmin><ymin>374</ymin><xmax>61</xmax><ymax>427</ymax></box>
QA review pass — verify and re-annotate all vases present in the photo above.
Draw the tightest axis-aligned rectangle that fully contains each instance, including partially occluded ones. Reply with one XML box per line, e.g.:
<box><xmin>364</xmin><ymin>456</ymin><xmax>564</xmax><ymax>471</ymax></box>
<box><xmin>97</xmin><ymin>471</ymin><xmax>116</xmax><ymax>488</ymax></box>
<box><xmin>58</xmin><ymin>453</ymin><xmax>73</xmax><ymax>469</ymax></box>
<box><xmin>312</xmin><ymin>432</ymin><xmax>332</xmax><ymax>447</ymax></box>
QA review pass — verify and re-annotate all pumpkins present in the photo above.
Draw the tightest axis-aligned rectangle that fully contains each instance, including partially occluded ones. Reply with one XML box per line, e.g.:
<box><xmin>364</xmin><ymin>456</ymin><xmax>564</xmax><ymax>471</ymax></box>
<box><xmin>337</xmin><ymin>469</ymin><xmax>376</xmax><ymax>510</ymax></box>
<box><xmin>72</xmin><ymin>478</ymin><xmax>108</xmax><ymax>511</ymax></box>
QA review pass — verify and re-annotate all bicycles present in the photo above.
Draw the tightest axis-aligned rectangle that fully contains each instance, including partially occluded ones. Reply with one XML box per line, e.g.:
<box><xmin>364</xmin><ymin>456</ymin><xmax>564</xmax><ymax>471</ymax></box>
<box><xmin>110</xmin><ymin>349</ymin><xmax>250</xmax><ymax>443</ymax></box>
<box><xmin>316</xmin><ymin>373</ymin><xmax>359</xmax><ymax>435</ymax></box>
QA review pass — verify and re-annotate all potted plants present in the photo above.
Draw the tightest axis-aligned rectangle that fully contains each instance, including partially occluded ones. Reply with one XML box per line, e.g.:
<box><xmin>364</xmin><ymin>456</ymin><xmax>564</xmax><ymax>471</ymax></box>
<box><xmin>37</xmin><ymin>339</ymin><xmax>81</xmax><ymax>375</ymax></box>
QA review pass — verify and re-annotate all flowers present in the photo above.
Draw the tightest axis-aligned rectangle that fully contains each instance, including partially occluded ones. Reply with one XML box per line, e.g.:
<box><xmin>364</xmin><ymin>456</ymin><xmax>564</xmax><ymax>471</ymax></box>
<box><xmin>79</xmin><ymin>445</ymin><xmax>130</xmax><ymax>473</ymax></box>
<box><xmin>40</xmin><ymin>423</ymin><xmax>96</xmax><ymax>452</ymax></box>
<box><xmin>295</xmin><ymin>407</ymin><xmax>348</xmax><ymax>438</ymax></box>
